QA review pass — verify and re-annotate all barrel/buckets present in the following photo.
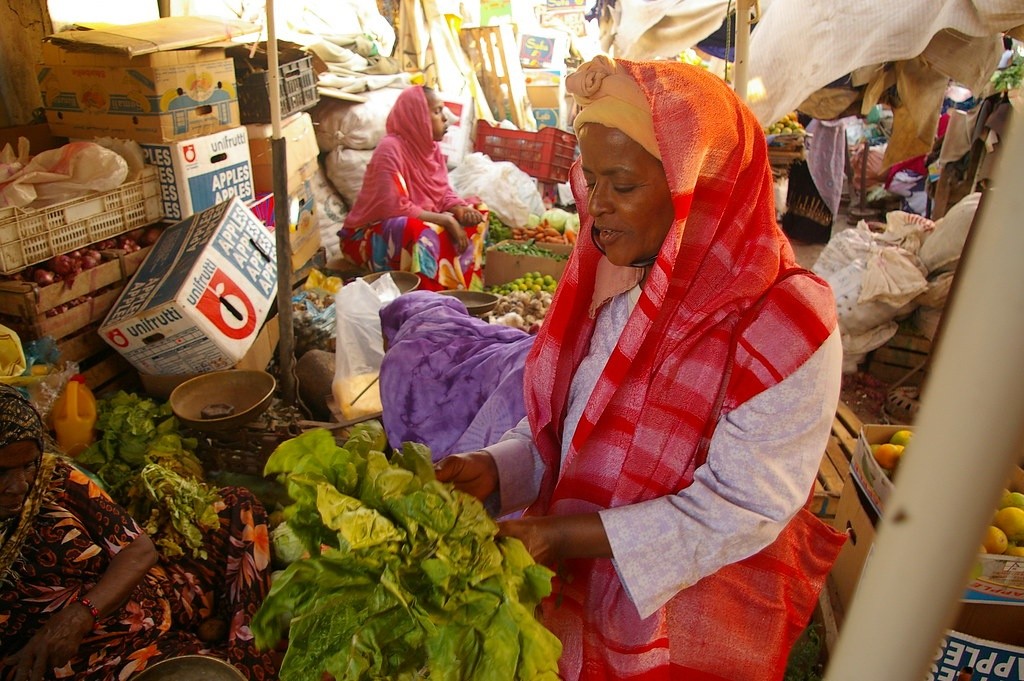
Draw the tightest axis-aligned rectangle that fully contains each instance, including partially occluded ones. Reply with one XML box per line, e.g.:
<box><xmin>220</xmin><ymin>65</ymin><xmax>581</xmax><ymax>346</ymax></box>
<box><xmin>51</xmin><ymin>374</ymin><xmax>97</xmax><ymax>458</ymax></box>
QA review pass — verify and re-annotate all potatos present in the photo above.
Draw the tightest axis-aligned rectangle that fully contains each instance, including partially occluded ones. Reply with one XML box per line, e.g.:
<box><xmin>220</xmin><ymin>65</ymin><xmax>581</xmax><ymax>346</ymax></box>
<box><xmin>197</xmin><ymin>618</ymin><xmax>226</xmax><ymax>642</ymax></box>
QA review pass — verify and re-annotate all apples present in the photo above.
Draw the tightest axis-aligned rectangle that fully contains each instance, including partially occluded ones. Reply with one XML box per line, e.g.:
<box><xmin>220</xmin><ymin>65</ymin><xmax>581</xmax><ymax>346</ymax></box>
<box><xmin>761</xmin><ymin>111</ymin><xmax>806</xmax><ymax>137</ymax></box>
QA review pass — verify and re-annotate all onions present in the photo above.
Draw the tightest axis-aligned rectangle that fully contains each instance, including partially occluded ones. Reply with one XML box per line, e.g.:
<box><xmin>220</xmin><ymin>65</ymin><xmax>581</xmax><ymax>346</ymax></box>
<box><xmin>0</xmin><ymin>228</ymin><xmax>163</xmax><ymax>327</ymax></box>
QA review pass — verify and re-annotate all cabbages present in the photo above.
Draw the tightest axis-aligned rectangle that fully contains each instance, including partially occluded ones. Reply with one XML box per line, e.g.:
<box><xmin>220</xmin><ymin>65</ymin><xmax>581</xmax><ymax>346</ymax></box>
<box><xmin>527</xmin><ymin>208</ymin><xmax>580</xmax><ymax>236</ymax></box>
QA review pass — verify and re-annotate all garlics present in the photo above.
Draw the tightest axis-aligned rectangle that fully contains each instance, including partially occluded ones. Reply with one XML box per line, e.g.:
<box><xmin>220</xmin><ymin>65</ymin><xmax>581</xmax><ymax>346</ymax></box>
<box><xmin>490</xmin><ymin>290</ymin><xmax>553</xmax><ymax>324</ymax></box>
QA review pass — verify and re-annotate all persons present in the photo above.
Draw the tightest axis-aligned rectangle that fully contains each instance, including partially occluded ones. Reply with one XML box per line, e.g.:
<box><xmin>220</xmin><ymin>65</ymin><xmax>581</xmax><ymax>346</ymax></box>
<box><xmin>866</xmin><ymin>104</ymin><xmax>893</xmax><ymax>145</ymax></box>
<box><xmin>436</xmin><ymin>52</ymin><xmax>852</xmax><ymax>681</ymax></box>
<box><xmin>378</xmin><ymin>290</ymin><xmax>545</xmax><ymax>519</ymax></box>
<box><xmin>997</xmin><ymin>38</ymin><xmax>1015</xmax><ymax>68</ymax></box>
<box><xmin>780</xmin><ymin>118</ymin><xmax>845</xmax><ymax>243</ymax></box>
<box><xmin>936</xmin><ymin>86</ymin><xmax>974</xmax><ymax>140</ymax></box>
<box><xmin>337</xmin><ymin>85</ymin><xmax>488</xmax><ymax>292</ymax></box>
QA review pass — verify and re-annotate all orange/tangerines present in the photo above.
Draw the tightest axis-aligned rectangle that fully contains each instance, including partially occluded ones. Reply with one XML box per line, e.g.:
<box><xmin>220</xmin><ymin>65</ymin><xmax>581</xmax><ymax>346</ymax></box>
<box><xmin>863</xmin><ymin>430</ymin><xmax>1024</xmax><ymax>560</ymax></box>
<box><xmin>485</xmin><ymin>271</ymin><xmax>557</xmax><ymax>295</ymax></box>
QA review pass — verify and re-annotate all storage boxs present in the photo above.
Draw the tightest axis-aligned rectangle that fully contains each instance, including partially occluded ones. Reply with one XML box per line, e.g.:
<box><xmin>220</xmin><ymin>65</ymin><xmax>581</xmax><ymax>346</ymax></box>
<box><xmin>832</xmin><ymin>474</ymin><xmax>1024</xmax><ymax>645</ymax></box>
<box><xmin>138</xmin><ymin>126</ymin><xmax>254</xmax><ymax>223</ymax></box>
<box><xmin>249</xmin><ymin>112</ymin><xmax>319</xmax><ymax>273</ymax></box>
<box><xmin>237</xmin><ymin>55</ymin><xmax>321</xmax><ymax>126</ymax></box>
<box><xmin>517</xmin><ymin>0</ymin><xmax>586</xmax><ymax>133</ymax></box>
<box><xmin>98</xmin><ymin>194</ymin><xmax>280</xmax><ymax>398</ymax></box>
<box><xmin>33</xmin><ymin>42</ymin><xmax>240</xmax><ymax>145</ymax></box>
<box><xmin>484</xmin><ymin>238</ymin><xmax>576</xmax><ymax>296</ymax></box>
<box><xmin>850</xmin><ymin>424</ymin><xmax>1024</xmax><ymax>607</ymax></box>
<box><xmin>473</xmin><ymin>119</ymin><xmax>576</xmax><ymax>186</ymax></box>
<box><xmin>0</xmin><ymin>162</ymin><xmax>165</xmax><ymax>275</ymax></box>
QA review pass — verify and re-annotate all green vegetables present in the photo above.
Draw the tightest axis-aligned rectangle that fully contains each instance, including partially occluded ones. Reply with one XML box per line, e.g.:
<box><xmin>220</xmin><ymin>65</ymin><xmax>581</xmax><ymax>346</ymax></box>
<box><xmin>72</xmin><ymin>390</ymin><xmax>219</xmax><ymax>560</ymax></box>
<box><xmin>989</xmin><ymin>46</ymin><xmax>1024</xmax><ymax>92</ymax></box>
<box><xmin>247</xmin><ymin>417</ymin><xmax>564</xmax><ymax>681</ymax></box>
<box><xmin>496</xmin><ymin>237</ymin><xmax>570</xmax><ymax>262</ymax></box>
<box><xmin>480</xmin><ymin>211</ymin><xmax>513</xmax><ymax>264</ymax></box>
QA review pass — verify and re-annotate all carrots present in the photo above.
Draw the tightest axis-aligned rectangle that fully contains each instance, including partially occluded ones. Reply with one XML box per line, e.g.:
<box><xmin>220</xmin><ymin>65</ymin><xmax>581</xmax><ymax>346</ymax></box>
<box><xmin>512</xmin><ymin>218</ymin><xmax>578</xmax><ymax>245</ymax></box>
